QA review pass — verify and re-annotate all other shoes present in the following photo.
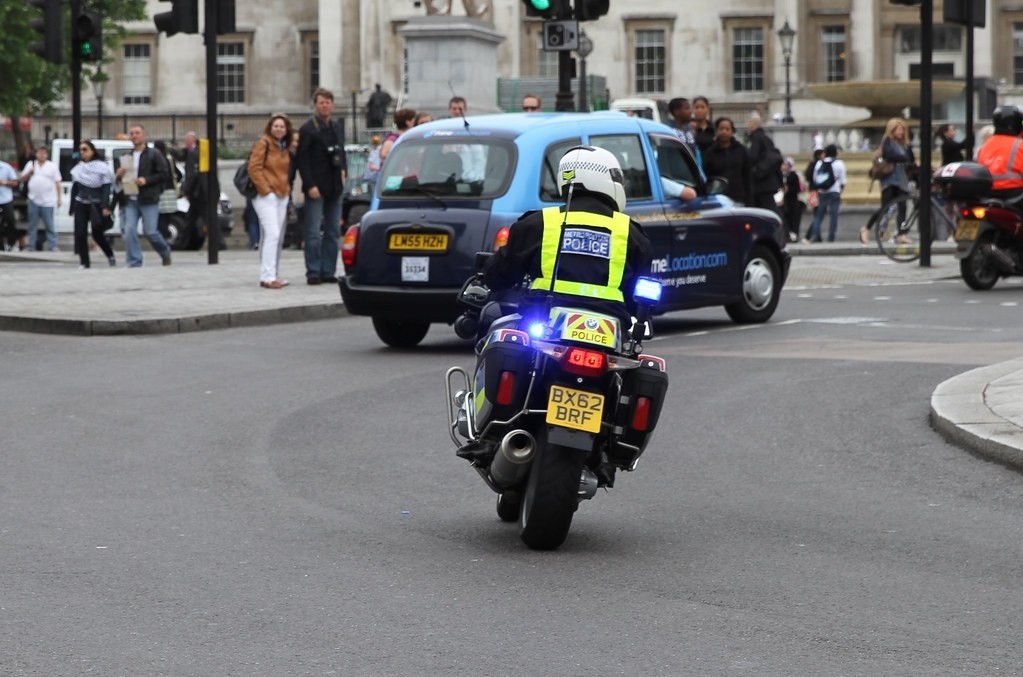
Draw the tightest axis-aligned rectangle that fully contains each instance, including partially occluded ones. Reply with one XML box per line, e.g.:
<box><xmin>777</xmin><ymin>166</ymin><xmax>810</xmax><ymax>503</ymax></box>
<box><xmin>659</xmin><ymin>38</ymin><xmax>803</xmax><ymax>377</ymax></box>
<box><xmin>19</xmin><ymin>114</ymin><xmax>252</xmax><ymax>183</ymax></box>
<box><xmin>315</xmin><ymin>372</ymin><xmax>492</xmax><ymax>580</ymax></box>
<box><xmin>78</xmin><ymin>265</ymin><xmax>84</xmax><ymax>269</ymax></box>
<box><xmin>323</xmin><ymin>277</ymin><xmax>337</xmax><ymax>283</ymax></box>
<box><xmin>108</xmin><ymin>256</ymin><xmax>115</xmax><ymax>266</ymax></box>
<box><xmin>260</xmin><ymin>279</ymin><xmax>281</xmax><ymax>289</ymax></box>
<box><xmin>309</xmin><ymin>276</ymin><xmax>321</xmax><ymax>285</ymax></box>
<box><xmin>161</xmin><ymin>252</ymin><xmax>171</xmax><ymax>266</ymax></box>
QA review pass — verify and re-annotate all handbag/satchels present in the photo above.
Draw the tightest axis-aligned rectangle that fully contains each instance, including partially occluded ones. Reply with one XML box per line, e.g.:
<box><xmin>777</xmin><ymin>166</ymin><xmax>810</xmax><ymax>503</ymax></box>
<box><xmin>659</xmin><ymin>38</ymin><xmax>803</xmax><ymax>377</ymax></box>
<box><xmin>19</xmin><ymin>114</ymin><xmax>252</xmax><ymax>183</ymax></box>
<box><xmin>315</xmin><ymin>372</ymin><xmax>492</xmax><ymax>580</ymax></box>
<box><xmin>159</xmin><ymin>188</ymin><xmax>178</xmax><ymax>214</ymax></box>
<box><xmin>868</xmin><ymin>160</ymin><xmax>894</xmax><ymax>178</ymax></box>
<box><xmin>19</xmin><ymin>160</ymin><xmax>34</xmax><ymax>197</ymax></box>
<box><xmin>234</xmin><ymin>139</ymin><xmax>270</xmax><ymax>198</ymax></box>
<box><xmin>751</xmin><ymin>131</ymin><xmax>783</xmax><ymax>180</ymax></box>
<box><xmin>90</xmin><ymin>202</ymin><xmax>114</xmax><ymax>233</ymax></box>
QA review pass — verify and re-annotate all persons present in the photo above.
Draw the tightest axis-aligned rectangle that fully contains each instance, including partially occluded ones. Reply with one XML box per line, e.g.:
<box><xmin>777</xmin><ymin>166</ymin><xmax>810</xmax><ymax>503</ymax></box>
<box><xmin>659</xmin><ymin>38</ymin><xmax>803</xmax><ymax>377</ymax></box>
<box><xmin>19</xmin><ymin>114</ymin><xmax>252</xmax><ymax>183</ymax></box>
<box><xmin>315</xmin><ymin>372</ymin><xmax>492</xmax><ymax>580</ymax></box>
<box><xmin>933</xmin><ymin>123</ymin><xmax>976</xmax><ymax>240</ymax></box>
<box><xmin>780</xmin><ymin>155</ymin><xmax>807</xmax><ymax>243</ymax></box>
<box><xmin>858</xmin><ymin>117</ymin><xmax>917</xmax><ymax>244</ymax></box>
<box><xmin>454</xmin><ymin>144</ymin><xmax>657</xmax><ymax>358</ymax></box>
<box><xmin>365</xmin><ymin>82</ymin><xmax>392</xmax><ymax>129</ymax></box>
<box><xmin>669</xmin><ymin>96</ymin><xmax>784</xmax><ymax>215</ymax></box>
<box><xmin>19</xmin><ymin>146</ymin><xmax>62</xmax><ymax>253</ymax></box>
<box><xmin>802</xmin><ymin>144</ymin><xmax>847</xmax><ymax>244</ymax></box>
<box><xmin>295</xmin><ymin>88</ymin><xmax>347</xmax><ymax>286</ymax></box>
<box><xmin>361</xmin><ymin>108</ymin><xmax>434</xmax><ymax>195</ymax></box>
<box><xmin>164</xmin><ymin>131</ymin><xmax>229</xmax><ymax>252</ymax></box>
<box><xmin>0</xmin><ymin>158</ymin><xmax>22</xmax><ymax>254</ymax></box>
<box><xmin>68</xmin><ymin>140</ymin><xmax>120</xmax><ymax>270</ymax></box>
<box><xmin>241</xmin><ymin>112</ymin><xmax>300</xmax><ymax>287</ymax></box>
<box><xmin>972</xmin><ymin>104</ymin><xmax>1023</xmax><ymax>223</ymax></box>
<box><xmin>521</xmin><ymin>95</ymin><xmax>544</xmax><ymax>114</ymax></box>
<box><xmin>447</xmin><ymin>97</ymin><xmax>467</xmax><ymax>118</ymax></box>
<box><xmin>104</xmin><ymin>124</ymin><xmax>172</xmax><ymax>267</ymax></box>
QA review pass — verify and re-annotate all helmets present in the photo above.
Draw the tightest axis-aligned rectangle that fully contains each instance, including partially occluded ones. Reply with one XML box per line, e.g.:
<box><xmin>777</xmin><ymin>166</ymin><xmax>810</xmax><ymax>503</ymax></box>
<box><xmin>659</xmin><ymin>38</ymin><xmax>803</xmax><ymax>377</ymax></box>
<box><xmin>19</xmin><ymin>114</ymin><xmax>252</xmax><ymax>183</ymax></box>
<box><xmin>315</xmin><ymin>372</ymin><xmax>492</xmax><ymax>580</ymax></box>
<box><xmin>993</xmin><ymin>103</ymin><xmax>1023</xmax><ymax>130</ymax></box>
<box><xmin>557</xmin><ymin>146</ymin><xmax>627</xmax><ymax>213</ymax></box>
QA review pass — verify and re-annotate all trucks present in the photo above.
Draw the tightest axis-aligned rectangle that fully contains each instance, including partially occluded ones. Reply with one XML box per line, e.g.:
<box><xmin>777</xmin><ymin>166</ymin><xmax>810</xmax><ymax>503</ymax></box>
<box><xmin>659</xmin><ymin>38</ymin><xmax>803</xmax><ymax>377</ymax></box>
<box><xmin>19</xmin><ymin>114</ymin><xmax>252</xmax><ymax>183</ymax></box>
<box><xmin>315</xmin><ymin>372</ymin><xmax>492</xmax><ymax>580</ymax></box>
<box><xmin>10</xmin><ymin>136</ymin><xmax>235</xmax><ymax>252</ymax></box>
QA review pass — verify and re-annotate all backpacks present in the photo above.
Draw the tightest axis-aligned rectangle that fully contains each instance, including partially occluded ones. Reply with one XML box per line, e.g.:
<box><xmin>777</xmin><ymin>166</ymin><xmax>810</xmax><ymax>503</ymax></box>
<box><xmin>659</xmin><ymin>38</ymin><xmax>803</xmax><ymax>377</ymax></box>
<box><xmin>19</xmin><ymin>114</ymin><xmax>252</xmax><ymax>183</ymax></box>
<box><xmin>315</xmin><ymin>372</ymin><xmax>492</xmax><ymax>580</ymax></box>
<box><xmin>815</xmin><ymin>158</ymin><xmax>837</xmax><ymax>189</ymax></box>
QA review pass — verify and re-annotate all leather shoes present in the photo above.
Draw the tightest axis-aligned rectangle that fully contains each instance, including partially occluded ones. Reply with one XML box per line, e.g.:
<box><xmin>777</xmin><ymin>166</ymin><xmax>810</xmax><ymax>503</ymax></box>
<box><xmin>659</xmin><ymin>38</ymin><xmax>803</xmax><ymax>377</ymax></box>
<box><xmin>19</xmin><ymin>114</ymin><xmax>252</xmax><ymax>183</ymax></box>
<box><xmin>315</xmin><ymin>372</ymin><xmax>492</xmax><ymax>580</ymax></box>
<box><xmin>455</xmin><ymin>441</ymin><xmax>494</xmax><ymax>461</ymax></box>
<box><xmin>600</xmin><ymin>463</ymin><xmax>616</xmax><ymax>488</ymax></box>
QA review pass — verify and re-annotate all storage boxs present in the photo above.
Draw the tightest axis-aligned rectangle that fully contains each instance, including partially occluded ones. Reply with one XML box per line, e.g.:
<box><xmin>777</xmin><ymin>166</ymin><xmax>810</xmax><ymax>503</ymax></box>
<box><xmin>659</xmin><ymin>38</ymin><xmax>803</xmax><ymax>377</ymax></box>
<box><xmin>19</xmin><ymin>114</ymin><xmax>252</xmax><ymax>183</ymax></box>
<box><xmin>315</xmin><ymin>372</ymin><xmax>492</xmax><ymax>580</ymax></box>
<box><xmin>618</xmin><ymin>362</ymin><xmax>672</xmax><ymax>455</ymax></box>
<box><xmin>483</xmin><ymin>341</ymin><xmax>535</xmax><ymax>415</ymax></box>
<box><xmin>929</xmin><ymin>161</ymin><xmax>995</xmax><ymax>201</ymax></box>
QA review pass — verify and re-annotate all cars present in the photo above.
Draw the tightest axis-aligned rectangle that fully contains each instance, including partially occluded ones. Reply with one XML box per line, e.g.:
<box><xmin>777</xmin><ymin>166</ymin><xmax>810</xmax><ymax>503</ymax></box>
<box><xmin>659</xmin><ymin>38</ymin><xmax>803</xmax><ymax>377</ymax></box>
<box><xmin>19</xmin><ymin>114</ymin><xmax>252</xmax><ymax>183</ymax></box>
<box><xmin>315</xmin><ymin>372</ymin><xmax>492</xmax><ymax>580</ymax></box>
<box><xmin>334</xmin><ymin>107</ymin><xmax>793</xmax><ymax>348</ymax></box>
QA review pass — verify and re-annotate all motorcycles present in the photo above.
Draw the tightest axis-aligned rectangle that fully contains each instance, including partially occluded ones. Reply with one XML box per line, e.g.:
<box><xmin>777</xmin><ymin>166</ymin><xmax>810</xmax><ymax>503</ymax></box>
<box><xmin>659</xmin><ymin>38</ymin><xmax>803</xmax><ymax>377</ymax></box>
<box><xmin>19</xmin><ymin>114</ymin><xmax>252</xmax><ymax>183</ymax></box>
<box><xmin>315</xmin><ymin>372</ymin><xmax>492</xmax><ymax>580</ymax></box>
<box><xmin>446</xmin><ymin>169</ymin><xmax>671</xmax><ymax>554</ymax></box>
<box><xmin>930</xmin><ymin>161</ymin><xmax>1023</xmax><ymax>290</ymax></box>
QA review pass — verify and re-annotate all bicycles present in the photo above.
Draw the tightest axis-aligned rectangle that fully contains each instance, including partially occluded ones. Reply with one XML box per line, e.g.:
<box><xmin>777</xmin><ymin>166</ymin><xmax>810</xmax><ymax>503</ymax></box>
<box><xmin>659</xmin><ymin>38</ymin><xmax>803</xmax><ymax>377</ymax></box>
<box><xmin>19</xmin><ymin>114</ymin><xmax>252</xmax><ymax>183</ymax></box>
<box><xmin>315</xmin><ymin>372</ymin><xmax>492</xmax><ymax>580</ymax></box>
<box><xmin>872</xmin><ymin>167</ymin><xmax>964</xmax><ymax>263</ymax></box>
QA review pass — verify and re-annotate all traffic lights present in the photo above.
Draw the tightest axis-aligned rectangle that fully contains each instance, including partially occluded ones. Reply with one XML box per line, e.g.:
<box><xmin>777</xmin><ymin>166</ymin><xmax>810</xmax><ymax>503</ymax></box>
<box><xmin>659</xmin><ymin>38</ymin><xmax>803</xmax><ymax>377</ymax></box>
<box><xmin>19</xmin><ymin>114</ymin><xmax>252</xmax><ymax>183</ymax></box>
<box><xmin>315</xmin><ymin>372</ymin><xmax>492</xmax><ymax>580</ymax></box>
<box><xmin>73</xmin><ymin>12</ymin><xmax>103</xmax><ymax>62</ymax></box>
<box><xmin>152</xmin><ymin>0</ymin><xmax>199</xmax><ymax>37</ymax></box>
<box><xmin>30</xmin><ymin>0</ymin><xmax>63</xmax><ymax>64</ymax></box>
<box><xmin>573</xmin><ymin>0</ymin><xmax>611</xmax><ymax>22</ymax></box>
<box><xmin>523</xmin><ymin>0</ymin><xmax>566</xmax><ymax>20</ymax></box>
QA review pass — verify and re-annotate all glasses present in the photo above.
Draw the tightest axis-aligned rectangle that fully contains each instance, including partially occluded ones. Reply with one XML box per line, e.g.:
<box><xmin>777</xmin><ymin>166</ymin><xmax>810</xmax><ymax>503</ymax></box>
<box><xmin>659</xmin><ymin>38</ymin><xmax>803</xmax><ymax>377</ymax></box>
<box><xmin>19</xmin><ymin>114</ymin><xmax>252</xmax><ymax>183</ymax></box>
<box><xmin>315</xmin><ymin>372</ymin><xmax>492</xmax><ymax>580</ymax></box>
<box><xmin>523</xmin><ymin>106</ymin><xmax>538</xmax><ymax>112</ymax></box>
<box><xmin>78</xmin><ymin>147</ymin><xmax>88</xmax><ymax>152</ymax></box>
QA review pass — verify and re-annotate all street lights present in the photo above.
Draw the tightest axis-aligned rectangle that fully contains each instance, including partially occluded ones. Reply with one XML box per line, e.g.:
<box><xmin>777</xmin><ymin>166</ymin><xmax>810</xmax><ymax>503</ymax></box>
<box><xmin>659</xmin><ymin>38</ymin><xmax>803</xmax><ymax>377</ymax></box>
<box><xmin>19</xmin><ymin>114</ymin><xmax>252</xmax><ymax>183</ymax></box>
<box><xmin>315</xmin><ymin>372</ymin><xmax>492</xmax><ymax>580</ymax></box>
<box><xmin>776</xmin><ymin>20</ymin><xmax>799</xmax><ymax>125</ymax></box>
<box><xmin>42</xmin><ymin>107</ymin><xmax>59</xmax><ymax>146</ymax></box>
<box><xmin>88</xmin><ymin>64</ymin><xmax>110</xmax><ymax>138</ymax></box>
